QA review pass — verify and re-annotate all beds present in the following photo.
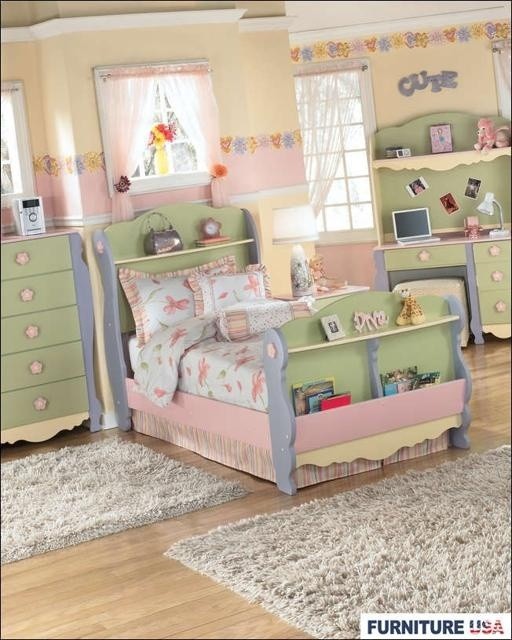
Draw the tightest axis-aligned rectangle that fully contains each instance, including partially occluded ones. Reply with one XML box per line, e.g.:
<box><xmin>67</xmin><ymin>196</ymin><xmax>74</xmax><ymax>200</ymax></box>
<box><xmin>92</xmin><ymin>203</ymin><xmax>472</xmax><ymax>495</ymax></box>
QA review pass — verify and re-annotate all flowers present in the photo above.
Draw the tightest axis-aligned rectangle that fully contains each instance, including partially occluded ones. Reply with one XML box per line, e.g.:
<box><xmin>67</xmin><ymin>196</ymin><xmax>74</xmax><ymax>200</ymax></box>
<box><xmin>210</xmin><ymin>164</ymin><xmax>228</xmax><ymax>180</ymax></box>
<box><xmin>146</xmin><ymin>119</ymin><xmax>177</xmax><ymax>150</ymax></box>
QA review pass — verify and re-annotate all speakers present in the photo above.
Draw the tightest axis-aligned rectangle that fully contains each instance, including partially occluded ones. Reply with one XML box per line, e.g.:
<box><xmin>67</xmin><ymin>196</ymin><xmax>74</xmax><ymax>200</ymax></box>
<box><xmin>11</xmin><ymin>196</ymin><xmax>46</xmax><ymax>236</ymax></box>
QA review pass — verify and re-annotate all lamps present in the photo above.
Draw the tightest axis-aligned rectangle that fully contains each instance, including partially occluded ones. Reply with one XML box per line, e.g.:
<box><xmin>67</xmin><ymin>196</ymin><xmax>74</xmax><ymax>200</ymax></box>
<box><xmin>270</xmin><ymin>206</ymin><xmax>319</xmax><ymax>297</ymax></box>
<box><xmin>476</xmin><ymin>192</ymin><xmax>509</xmax><ymax>238</ymax></box>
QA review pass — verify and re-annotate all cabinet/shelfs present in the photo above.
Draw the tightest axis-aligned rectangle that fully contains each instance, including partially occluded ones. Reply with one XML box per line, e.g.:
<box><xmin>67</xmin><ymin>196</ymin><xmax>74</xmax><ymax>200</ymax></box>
<box><xmin>370</xmin><ymin>112</ymin><xmax>511</xmax><ymax>344</ymax></box>
<box><xmin>1</xmin><ymin>230</ymin><xmax>102</xmax><ymax>445</ymax></box>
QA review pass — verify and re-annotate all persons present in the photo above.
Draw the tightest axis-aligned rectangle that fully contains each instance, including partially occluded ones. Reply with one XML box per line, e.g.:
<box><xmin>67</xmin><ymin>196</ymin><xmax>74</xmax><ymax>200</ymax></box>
<box><xmin>474</xmin><ymin>118</ymin><xmax>511</xmax><ymax>150</ymax></box>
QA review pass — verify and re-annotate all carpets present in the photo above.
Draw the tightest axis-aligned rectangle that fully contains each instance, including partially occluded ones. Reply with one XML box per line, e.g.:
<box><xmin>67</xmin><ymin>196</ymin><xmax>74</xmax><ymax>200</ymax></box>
<box><xmin>0</xmin><ymin>435</ymin><xmax>249</xmax><ymax>566</ymax></box>
<box><xmin>163</xmin><ymin>445</ymin><xmax>511</xmax><ymax>639</ymax></box>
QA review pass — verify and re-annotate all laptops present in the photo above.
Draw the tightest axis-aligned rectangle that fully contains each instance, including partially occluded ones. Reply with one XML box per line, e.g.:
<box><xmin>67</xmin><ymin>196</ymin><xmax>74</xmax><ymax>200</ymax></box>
<box><xmin>391</xmin><ymin>208</ymin><xmax>441</xmax><ymax>246</ymax></box>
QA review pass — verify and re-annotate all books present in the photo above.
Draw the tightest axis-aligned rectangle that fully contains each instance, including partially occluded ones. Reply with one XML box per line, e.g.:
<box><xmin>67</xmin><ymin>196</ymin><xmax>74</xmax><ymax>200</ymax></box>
<box><xmin>292</xmin><ymin>376</ymin><xmax>352</xmax><ymax>416</ymax></box>
<box><xmin>194</xmin><ymin>235</ymin><xmax>230</xmax><ymax>247</ymax></box>
<box><xmin>380</xmin><ymin>365</ymin><xmax>441</xmax><ymax>396</ymax></box>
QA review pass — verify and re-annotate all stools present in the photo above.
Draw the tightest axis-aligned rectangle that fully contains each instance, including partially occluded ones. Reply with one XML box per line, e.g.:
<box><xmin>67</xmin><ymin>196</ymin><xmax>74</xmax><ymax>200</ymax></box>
<box><xmin>393</xmin><ymin>276</ymin><xmax>469</xmax><ymax>348</ymax></box>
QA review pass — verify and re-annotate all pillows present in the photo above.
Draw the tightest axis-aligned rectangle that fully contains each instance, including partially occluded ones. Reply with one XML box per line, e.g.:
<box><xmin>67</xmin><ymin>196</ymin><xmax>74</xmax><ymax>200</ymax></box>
<box><xmin>216</xmin><ymin>297</ymin><xmax>317</xmax><ymax>343</ymax></box>
<box><xmin>118</xmin><ymin>255</ymin><xmax>237</xmax><ymax>347</ymax></box>
<box><xmin>187</xmin><ymin>263</ymin><xmax>273</xmax><ymax>316</ymax></box>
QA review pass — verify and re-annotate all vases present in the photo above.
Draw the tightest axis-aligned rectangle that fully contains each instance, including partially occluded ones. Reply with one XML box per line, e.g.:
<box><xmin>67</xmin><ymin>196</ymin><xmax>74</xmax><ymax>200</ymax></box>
<box><xmin>157</xmin><ymin>145</ymin><xmax>169</xmax><ymax>175</ymax></box>
<box><xmin>211</xmin><ymin>179</ymin><xmax>225</xmax><ymax>208</ymax></box>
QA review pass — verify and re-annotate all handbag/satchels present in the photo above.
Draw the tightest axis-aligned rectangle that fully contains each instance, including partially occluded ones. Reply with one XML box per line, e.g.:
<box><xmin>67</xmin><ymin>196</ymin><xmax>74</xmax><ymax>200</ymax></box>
<box><xmin>145</xmin><ymin>228</ymin><xmax>183</xmax><ymax>255</ymax></box>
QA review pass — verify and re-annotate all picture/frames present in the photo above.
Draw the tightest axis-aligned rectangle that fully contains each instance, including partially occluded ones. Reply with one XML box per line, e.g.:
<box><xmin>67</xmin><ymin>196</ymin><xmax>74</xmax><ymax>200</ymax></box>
<box><xmin>430</xmin><ymin>124</ymin><xmax>453</xmax><ymax>154</ymax></box>
<box><xmin>320</xmin><ymin>314</ymin><xmax>346</xmax><ymax>342</ymax></box>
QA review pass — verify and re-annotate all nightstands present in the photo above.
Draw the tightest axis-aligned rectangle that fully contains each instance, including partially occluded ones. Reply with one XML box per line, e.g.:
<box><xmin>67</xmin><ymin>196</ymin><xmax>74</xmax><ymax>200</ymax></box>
<box><xmin>272</xmin><ymin>286</ymin><xmax>370</xmax><ymax>310</ymax></box>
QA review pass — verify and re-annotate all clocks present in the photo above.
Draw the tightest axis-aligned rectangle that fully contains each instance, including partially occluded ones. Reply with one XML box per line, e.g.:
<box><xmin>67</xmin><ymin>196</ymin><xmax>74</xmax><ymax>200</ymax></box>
<box><xmin>200</xmin><ymin>216</ymin><xmax>222</xmax><ymax>239</ymax></box>
